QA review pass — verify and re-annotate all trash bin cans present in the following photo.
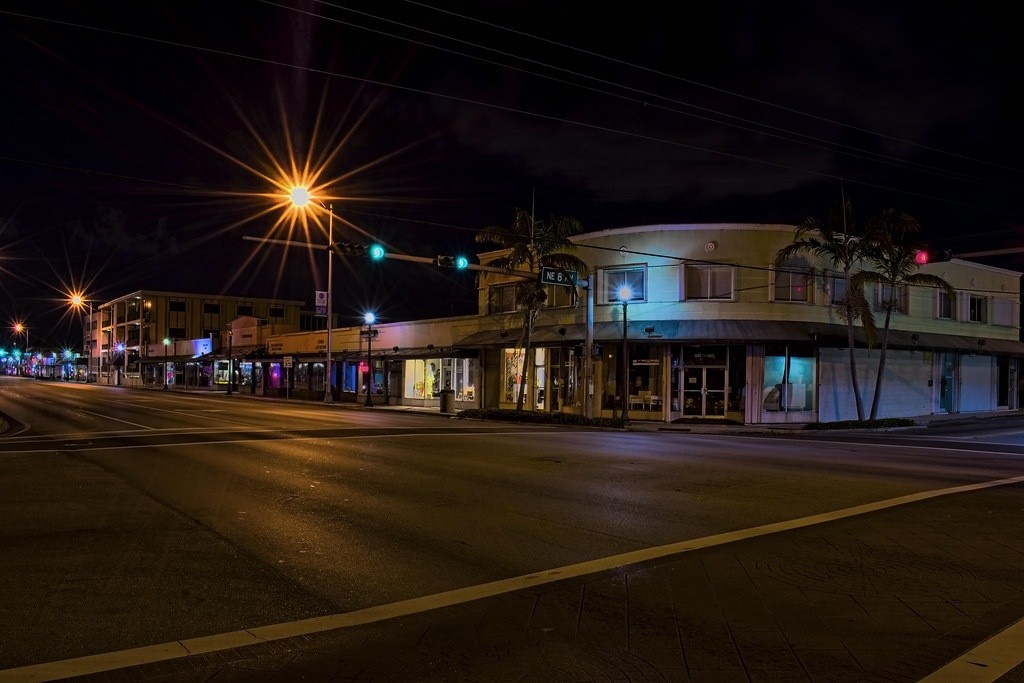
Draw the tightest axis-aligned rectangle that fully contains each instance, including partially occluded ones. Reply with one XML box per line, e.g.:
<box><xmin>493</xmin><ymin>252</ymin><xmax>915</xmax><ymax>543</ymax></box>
<box><xmin>440</xmin><ymin>390</ymin><xmax>455</xmax><ymax>413</ymax></box>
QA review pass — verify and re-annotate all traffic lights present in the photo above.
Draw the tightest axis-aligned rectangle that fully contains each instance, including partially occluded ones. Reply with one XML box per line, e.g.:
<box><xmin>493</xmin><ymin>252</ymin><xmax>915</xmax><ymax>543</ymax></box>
<box><xmin>437</xmin><ymin>255</ymin><xmax>468</xmax><ymax>269</ymax></box>
<box><xmin>916</xmin><ymin>249</ymin><xmax>951</xmax><ymax>264</ymax></box>
<box><xmin>330</xmin><ymin>242</ymin><xmax>384</xmax><ymax>260</ymax></box>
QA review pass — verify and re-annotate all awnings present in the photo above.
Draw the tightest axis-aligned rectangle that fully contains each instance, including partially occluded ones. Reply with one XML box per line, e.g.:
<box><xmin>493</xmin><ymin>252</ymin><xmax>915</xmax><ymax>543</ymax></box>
<box><xmin>199</xmin><ymin>344</ymin><xmax>477</xmax><ymax>361</ymax></box>
<box><xmin>452</xmin><ymin>320</ymin><xmax>1023</xmax><ymax>357</ymax></box>
<box><xmin>134</xmin><ymin>355</ymin><xmax>211</xmax><ymax>363</ymax></box>
<box><xmin>107</xmin><ymin>354</ymin><xmax>124</xmax><ymax>365</ymax></box>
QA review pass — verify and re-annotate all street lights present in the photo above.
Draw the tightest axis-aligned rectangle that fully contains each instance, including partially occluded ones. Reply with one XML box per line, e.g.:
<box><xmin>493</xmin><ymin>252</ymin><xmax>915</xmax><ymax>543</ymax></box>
<box><xmin>117</xmin><ymin>344</ymin><xmax>123</xmax><ymax>386</ymax></box>
<box><xmin>163</xmin><ymin>338</ymin><xmax>171</xmax><ymax>390</ymax></box>
<box><xmin>14</xmin><ymin>324</ymin><xmax>29</xmax><ymax>377</ymax></box>
<box><xmin>72</xmin><ymin>296</ymin><xmax>93</xmax><ymax>383</ymax></box>
<box><xmin>617</xmin><ymin>285</ymin><xmax>633</xmax><ymax>427</ymax></box>
<box><xmin>289</xmin><ymin>184</ymin><xmax>333</xmax><ymax>404</ymax></box>
<box><xmin>364</xmin><ymin>312</ymin><xmax>376</xmax><ymax>408</ymax></box>
